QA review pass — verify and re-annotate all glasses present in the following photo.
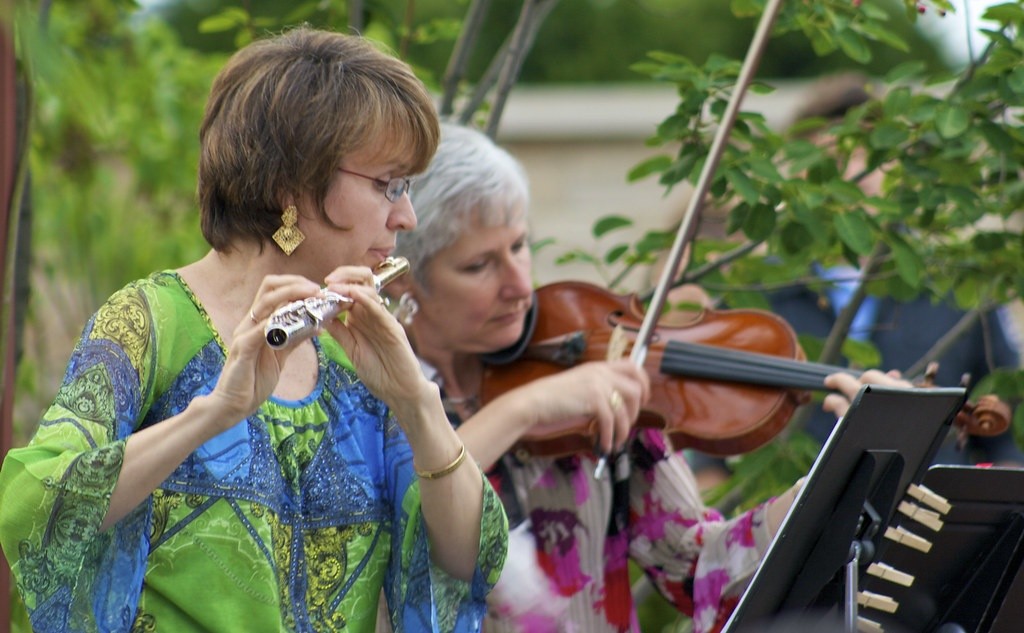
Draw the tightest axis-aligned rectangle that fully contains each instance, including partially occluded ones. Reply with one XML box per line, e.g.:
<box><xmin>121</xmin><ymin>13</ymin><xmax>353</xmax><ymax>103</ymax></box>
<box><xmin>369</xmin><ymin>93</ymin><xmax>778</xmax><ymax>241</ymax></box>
<box><xmin>337</xmin><ymin>167</ymin><xmax>416</xmax><ymax>202</ymax></box>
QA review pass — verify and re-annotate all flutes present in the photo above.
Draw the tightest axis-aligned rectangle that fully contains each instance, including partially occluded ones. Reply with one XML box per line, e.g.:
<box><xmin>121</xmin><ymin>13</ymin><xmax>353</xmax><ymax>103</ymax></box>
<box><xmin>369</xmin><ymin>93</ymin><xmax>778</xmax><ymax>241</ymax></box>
<box><xmin>264</xmin><ymin>256</ymin><xmax>411</xmax><ymax>351</ymax></box>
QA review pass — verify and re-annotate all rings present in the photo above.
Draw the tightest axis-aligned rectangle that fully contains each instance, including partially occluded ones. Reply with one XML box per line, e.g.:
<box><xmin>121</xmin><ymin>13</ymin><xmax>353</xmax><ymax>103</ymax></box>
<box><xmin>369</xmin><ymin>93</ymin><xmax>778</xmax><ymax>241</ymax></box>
<box><xmin>249</xmin><ymin>309</ymin><xmax>260</xmax><ymax>324</ymax></box>
<box><xmin>610</xmin><ymin>392</ymin><xmax>622</xmax><ymax>408</ymax></box>
<box><xmin>380</xmin><ymin>296</ymin><xmax>390</xmax><ymax>306</ymax></box>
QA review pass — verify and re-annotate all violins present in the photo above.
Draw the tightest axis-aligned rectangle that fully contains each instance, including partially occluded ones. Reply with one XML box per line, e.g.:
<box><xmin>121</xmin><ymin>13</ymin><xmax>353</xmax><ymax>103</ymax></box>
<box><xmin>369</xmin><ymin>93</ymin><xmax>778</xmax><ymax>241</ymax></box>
<box><xmin>478</xmin><ymin>281</ymin><xmax>1014</xmax><ymax>456</ymax></box>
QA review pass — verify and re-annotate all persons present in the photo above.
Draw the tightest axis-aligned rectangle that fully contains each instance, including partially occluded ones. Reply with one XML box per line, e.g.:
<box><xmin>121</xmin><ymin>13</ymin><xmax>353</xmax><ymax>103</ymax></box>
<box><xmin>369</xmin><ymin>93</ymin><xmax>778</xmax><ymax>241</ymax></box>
<box><xmin>0</xmin><ymin>26</ymin><xmax>508</xmax><ymax>633</ymax></box>
<box><xmin>374</xmin><ymin>123</ymin><xmax>915</xmax><ymax>633</ymax></box>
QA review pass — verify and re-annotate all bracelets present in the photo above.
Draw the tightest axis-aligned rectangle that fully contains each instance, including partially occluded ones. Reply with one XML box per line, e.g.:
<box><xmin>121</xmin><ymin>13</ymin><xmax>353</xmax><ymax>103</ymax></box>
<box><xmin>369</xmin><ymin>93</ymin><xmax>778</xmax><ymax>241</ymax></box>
<box><xmin>413</xmin><ymin>445</ymin><xmax>466</xmax><ymax>480</ymax></box>
